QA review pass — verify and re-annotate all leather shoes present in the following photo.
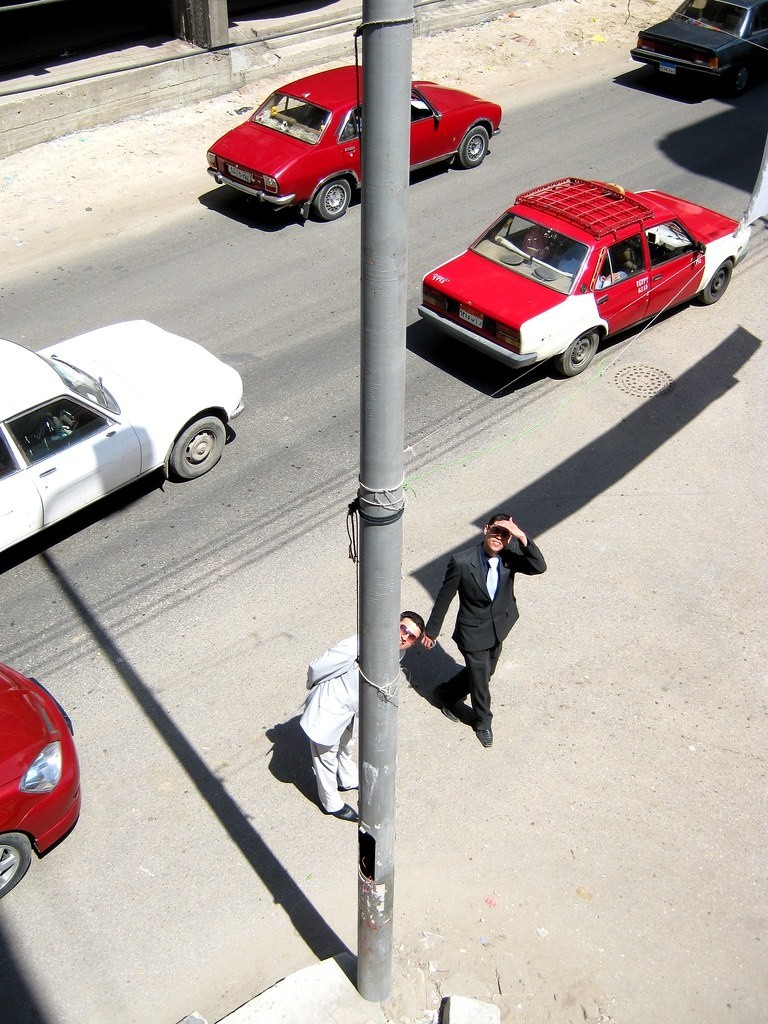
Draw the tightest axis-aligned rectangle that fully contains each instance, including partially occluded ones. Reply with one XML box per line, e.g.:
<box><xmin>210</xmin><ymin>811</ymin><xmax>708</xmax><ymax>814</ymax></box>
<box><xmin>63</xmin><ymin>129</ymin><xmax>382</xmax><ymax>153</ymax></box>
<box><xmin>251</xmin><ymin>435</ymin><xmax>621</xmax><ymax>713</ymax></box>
<box><xmin>432</xmin><ymin>689</ymin><xmax>463</xmax><ymax>722</ymax></box>
<box><xmin>476</xmin><ymin>728</ymin><xmax>493</xmax><ymax>747</ymax></box>
<box><xmin>332</xmin><ymin>803</ymin><xmax>359</xmax><ymax>822</ymax></box>
<box><xmin>338</xmin><ymin>784</ymin><xmax>360</xmax><ymax>791</ymax></box>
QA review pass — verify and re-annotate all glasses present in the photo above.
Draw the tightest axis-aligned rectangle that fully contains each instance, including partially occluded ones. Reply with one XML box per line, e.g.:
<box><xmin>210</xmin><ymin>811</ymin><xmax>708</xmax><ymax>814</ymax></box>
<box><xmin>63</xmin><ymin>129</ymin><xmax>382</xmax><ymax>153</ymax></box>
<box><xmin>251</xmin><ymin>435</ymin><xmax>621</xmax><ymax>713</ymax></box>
<box><xmin>488</xmin><ymin>527</ymin><xmax>510</xmax><ymax>538</ymax></box>
<box><xmin>400</xmin><ymin>624</ymin><xmax>416</xmax><ymax>641</ymax></box>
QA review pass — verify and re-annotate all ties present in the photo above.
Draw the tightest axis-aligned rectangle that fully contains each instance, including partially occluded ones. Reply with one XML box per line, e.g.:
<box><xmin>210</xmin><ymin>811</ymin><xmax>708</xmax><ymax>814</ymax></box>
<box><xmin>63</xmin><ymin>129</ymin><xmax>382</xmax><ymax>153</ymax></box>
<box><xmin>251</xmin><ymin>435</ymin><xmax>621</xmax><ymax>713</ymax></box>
<box><xmin>486</xmin><ymin>557</ymin><xmax>499</xmax><ymax>602</ymax></box>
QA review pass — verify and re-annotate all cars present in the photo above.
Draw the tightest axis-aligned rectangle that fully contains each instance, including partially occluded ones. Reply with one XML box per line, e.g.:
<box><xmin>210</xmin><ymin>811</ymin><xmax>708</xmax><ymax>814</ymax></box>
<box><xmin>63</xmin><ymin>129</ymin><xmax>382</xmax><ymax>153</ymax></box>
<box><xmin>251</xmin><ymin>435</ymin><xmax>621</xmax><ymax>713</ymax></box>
<box><xmin>630</xmin><ymin>0</ymin><xmax>768</xmax><ymax>97</ymax></box>
<box><xmin>203</xmin><ymin>64</ymin><xmax>503</xmax><ymax>225</ymax></box>
<box><xmin>417</xmin><ymin>175</ymin><xmax>753</xmax><ymax>378</ymax></box>
<box><xmin>0</xmin><ymin>318</ymin><xmax>244</xmax><ymax>555</ymax></box>
<box><xmin>0</xmin><ymin>664</ymin><xmax>82</xmax><ymax>899</ymax></box>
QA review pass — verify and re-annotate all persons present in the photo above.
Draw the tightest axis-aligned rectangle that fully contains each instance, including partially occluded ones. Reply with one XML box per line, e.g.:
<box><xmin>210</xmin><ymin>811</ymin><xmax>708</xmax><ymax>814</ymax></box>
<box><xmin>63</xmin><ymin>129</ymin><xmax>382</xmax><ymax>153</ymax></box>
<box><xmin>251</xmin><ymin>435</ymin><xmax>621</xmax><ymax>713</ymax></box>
<box><xmin>420</xmin><ymin>513</ymin><xmax>548</xmax><ymax>748</ymax></box>
<box><xmin>299</xmin><ymin>610</ymin><xmax>425</xmax><ymax>822</ymax></box>
